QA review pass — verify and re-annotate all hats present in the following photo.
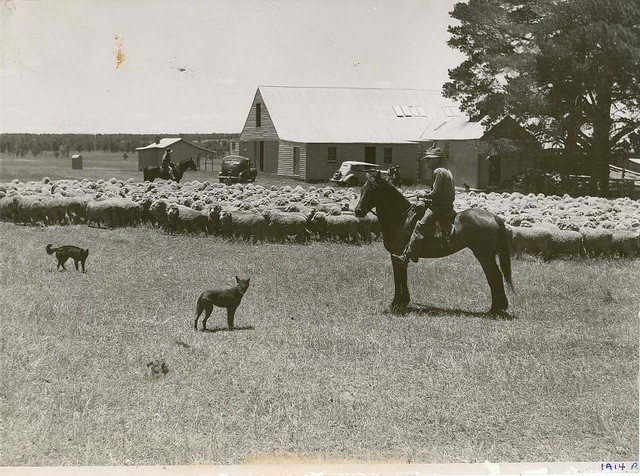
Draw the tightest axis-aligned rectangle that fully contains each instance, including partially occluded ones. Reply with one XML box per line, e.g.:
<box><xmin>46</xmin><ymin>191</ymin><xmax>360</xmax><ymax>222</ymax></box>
<box><xmin>421</xmin><ymin>146</ymin><xmax>447</xmax><ymax>161</ymax></box>
<box><xmin>165</xmin><ymin>148</ymin><xmax>173</xmax><ymax>153</ymax></box>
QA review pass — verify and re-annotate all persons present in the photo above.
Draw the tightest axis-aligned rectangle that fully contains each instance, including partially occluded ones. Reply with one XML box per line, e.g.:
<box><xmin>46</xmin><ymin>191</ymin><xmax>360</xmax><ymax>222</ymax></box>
<box><xmin>391</xmin><ymin>146</ymin><xmax>455</xmax><ymax>271</ymax></box>
<box><xmin>159</xmin><ymin>146</ymin><xmax>180</xmax><ymax>182</ymax></box>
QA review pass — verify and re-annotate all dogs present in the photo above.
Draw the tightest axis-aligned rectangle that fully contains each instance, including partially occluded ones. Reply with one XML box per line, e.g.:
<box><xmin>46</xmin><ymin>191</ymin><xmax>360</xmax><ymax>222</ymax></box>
<box><xmin>195</xmin><ymin>275</ymin><xmax>251</xmax><ymax>332</ymax></box>
<box><xmin>46</xmin><ymin>244</ymin><xmax>88</xmax><ymax>271</ymax></box>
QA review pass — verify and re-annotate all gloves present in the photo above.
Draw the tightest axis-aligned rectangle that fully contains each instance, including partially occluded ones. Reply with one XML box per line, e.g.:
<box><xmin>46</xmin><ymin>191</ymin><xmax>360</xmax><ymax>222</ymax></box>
<box><xmin>424</xmin><ymin>189</ymin><xmax>430</xmax><ymax>193</ymax></box>
<box><xmin>417</xmin><ymin>190</ymin><xmax>427</xmax><ymax>197</ymax></box>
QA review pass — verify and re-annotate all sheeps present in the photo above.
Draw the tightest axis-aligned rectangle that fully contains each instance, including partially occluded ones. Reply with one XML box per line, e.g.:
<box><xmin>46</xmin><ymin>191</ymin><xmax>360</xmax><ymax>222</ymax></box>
<box><xmin>1</xmin><ymin>178</ymin><xmax>383</xmax><ymax>245</ymax></box>
<box><xmin>400</xmin><ymin>188</ymin><xmax>430</xmax><ymax>204</ymax></box>
<box><xmin>455</xmin><ymin>191</ymin><xmax>640</xmax><ymax>264</ymax></box>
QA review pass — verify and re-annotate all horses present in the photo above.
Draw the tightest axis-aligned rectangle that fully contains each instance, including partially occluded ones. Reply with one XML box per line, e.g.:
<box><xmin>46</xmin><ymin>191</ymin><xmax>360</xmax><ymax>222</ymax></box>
<box><xmin>356</xmin><ymin>171</ymin><xmax>512</xmax><ymax>314</ymax></box>
<box><xmin>144</xmin><ymin>158</ymin><xmax>197</xmax><ymax>183</ymax></box>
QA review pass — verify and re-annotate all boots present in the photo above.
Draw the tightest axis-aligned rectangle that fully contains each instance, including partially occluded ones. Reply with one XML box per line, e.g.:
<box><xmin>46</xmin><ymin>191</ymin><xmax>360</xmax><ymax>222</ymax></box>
<box><xmin>392</xmin><ymin>221</ymin><xmax>425</xmax><ymax>267</ymax></box>
